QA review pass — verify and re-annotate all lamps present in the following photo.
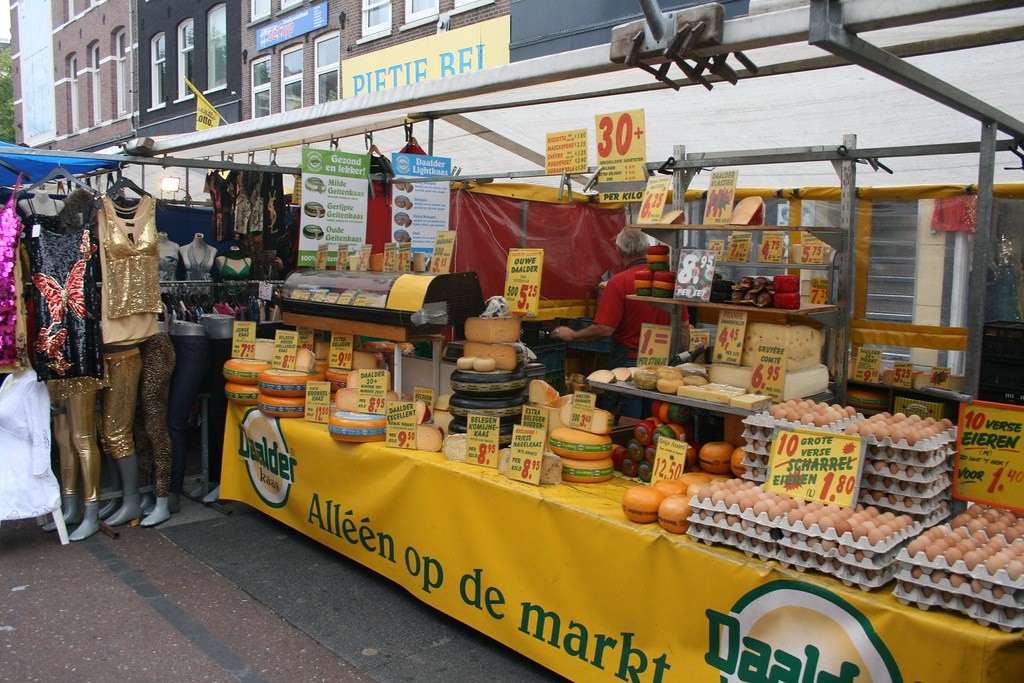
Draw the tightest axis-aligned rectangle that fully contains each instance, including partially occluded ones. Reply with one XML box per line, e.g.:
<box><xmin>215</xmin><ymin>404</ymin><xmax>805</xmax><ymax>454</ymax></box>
<box><xmin>159</xmin><ymin>175</ymin><xmax>181</xmax><ymax>199</ymax></box>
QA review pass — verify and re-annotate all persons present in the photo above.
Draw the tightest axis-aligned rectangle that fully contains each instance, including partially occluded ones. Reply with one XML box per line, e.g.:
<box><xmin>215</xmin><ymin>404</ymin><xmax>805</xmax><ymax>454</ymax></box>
<box><xmin>19</xmin><ymin>190</ymin><xmax>66</xmax><ymax>281</ymax></box>
<box><xmin>139</xmin><ymin>331</ymin><xmax>173</xmax><ymax>526</ymax></box>
<box><xmin>43</xmin><ymin>376</ymin><xmax>101</xmax><ymax>541</ymax></box>
<box><xmin>0</xmin><ymin>358</ymin><xmax>62</xmax><ymax>521</ymax></box>
<box><xmin>96</xmin><ymin>347</ymin><xmax>142</xmax><ymax>526</ymax></box>
<box><xmin>215</xmin><ymin>246</ymin><xmax>251</xmax><ymax>281</ymax></box>
<box><xmin>191</xmin><ymin>313</ymin><xmax>235</xmax><ymax>501</ymax></box>
<box><xmin>549</xmin><ymin>227</ymin><xmax>690</xmax><ymax>420</ymax></box>
<box><xmin>139</xmin><ymin>320</ymin><xmax>210</xmax><ymax>516</ymax></box>
<box><xmin>158</xmin><ymin>232</ymin><xmax>179</xmax><ymax>282</ymax></box>
<box><xmin>178</xmin><ymin>233</ymin><xmax>218</xmax><ymax>280</ymax></box>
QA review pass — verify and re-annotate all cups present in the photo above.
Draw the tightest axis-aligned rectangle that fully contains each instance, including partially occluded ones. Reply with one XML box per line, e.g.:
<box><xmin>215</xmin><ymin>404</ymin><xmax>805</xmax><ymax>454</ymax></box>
<box><xmin>349</xmin><ymin>256</ymin><xmax>360</xmax><ymax>271</ymax></box>
<box><xmin>413</xmin><ymin>252</ymin><xmax>426</xmax><ymax>272</ymax></box>
<box><xmin>370</xmin><ymin>253</ymin><xmax>384</xmax><ymax>272</ymax></box>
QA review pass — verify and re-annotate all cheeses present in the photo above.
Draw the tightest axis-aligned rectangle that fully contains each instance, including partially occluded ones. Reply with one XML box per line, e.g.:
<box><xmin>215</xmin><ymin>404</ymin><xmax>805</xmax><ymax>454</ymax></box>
<box><xmin>222</xmin><ymin>197</ymin><xmax>831</xmax><ymax>533</ymax></box>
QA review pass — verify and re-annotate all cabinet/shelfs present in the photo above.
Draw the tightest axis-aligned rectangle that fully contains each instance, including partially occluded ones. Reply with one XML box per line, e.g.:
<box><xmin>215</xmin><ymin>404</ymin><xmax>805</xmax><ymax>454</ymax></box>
<box><xmin>587</xmin><ymin>133</ymin><xmax>855</xmax><ymax>476</ymax></box>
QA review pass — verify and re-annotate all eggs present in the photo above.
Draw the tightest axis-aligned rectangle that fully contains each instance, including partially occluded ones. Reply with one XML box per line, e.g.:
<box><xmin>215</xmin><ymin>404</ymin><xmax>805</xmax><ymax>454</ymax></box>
<box><xmin>696</xmin><ymin>396</ymin><xmax>1024</xmax><ymax>620</ymax></box>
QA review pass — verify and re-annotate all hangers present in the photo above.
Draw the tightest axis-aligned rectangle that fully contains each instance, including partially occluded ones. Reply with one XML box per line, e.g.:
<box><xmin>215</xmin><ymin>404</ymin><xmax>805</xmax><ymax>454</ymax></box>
<box><xmin>368</xmin><ymin>135</ymin><xmax>381</xmax><ymax>156</ymax></box>
<box><xmin>407</xmin><ymin>136</ymin><xmax>419</xmax><ymax>149</ymax></box>
<box><xmin>271</xmin><ymin>160</ymin><xmax>278</xmax><ymax>166</ymax></box>
<box><xmin>16</xmin><ymin>161</ymin><xmax>166</xmax><ymax>213</ymax></box>
<box><xmin>157</xmin><ymin>276</ymin><xmax>285</xmax><ymax>320</ymax></box>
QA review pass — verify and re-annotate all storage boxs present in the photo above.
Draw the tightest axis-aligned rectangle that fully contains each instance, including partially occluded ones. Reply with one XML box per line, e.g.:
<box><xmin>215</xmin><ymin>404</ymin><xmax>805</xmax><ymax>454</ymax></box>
<box><xmin>528</xmin><ymin>343</ymin><xmax>567</xmax><ymax>393</ymax></box>
<box><xmin>555</xmin><ymin>315</ymin><xmax>619</xmax><ymax>353</ymax></box>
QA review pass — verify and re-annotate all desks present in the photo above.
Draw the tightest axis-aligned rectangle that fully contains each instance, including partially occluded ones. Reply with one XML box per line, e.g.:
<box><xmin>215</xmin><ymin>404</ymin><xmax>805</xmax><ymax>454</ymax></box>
<box><xmin>218</xmin><ymin>392</ymin><xmax>1024</xmax><ymax>683</ymax></box>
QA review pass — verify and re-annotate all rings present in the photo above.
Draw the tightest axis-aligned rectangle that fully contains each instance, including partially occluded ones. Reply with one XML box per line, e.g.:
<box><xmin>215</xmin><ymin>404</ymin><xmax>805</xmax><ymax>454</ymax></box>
<box><xmin>557</xmin><ymin>329</ymin><xmax>558</xmax><ymax>332</ymax></box>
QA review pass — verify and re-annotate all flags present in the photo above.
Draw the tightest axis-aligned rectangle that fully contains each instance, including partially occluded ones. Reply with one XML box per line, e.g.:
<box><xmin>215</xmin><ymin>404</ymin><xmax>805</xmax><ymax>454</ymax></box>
<box><xmin>186</xmin><ymin>82</ymin><xmax>220</xmax><ymax>131</ymax></box>
